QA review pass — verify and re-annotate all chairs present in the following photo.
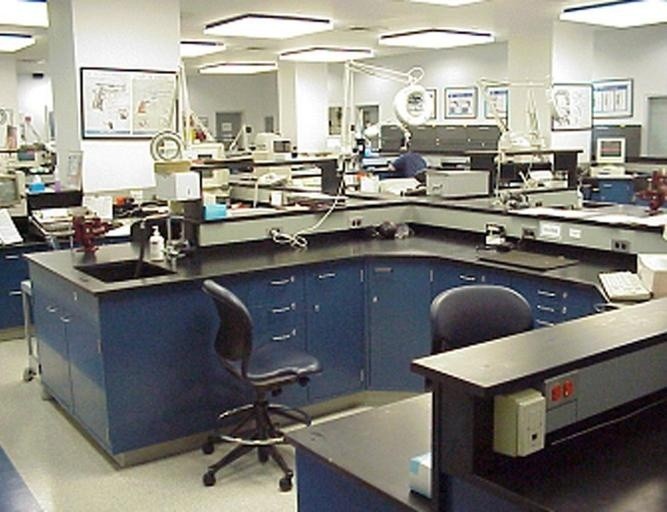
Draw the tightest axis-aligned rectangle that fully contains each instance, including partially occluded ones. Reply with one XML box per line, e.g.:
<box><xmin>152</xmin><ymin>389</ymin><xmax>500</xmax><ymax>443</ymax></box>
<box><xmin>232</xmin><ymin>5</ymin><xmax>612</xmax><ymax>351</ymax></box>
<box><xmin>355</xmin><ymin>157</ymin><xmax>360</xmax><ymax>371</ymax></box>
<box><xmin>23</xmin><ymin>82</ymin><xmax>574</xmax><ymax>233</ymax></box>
<box><xmin>204</xmin><ymin>279</ymin><xmax>323</xmax><ymax>490</ymax></box>
<box><xmin>426</xmin><ymin>283</ymin><xmax>535</xmax><ymax>393</ymax></box>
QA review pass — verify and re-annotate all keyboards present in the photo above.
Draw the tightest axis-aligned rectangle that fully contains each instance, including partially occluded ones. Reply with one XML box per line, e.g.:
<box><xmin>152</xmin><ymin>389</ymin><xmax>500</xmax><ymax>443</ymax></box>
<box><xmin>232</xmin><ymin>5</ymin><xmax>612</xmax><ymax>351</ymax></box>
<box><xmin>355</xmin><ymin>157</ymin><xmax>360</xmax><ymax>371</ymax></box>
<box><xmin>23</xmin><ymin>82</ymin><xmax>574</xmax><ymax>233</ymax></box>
<box><xmin>598</xmin><ymin>272</ymin><xmax>649</xmax><ymax>300</ymax></box>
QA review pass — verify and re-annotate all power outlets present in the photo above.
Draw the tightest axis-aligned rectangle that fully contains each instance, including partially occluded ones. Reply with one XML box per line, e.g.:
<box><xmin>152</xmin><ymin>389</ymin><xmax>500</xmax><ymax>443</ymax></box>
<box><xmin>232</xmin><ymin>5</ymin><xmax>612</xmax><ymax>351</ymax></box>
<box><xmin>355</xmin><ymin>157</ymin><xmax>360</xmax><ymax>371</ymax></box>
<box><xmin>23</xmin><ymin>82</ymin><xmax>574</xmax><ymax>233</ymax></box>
<box><xmin>492</xmin><ymin>391</ymin><xmax>545</xmax><ymax>457</ymax></box>
<box><xmin>350</xmin><ymin>217</ymin><xmax>363</xmax><ymax>229</ymax></box>
<box><xmin>270</xmin><ymin>227</ymin><xmax>284</xmax><ymax>238</ymax></box>
<box><xmin>521</xmin><ymin>226</ymin><xmax>538</xmax><ymax>241</ymax></box>
<box><xmin>613</xmin><ymin>239</ymin><xmax>630</xmax><ymax>253</ymax></box>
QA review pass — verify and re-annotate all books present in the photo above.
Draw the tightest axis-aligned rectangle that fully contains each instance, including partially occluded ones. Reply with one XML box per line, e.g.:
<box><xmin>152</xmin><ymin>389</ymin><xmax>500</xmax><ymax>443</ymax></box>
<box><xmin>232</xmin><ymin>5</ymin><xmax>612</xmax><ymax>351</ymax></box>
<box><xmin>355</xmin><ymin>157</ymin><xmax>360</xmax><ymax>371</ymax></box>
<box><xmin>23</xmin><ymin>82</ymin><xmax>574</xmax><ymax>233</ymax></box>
<box><xmin>598</xmin><ymin>271</ymin><xmax>652</xmax><ymax>302</ymax></box>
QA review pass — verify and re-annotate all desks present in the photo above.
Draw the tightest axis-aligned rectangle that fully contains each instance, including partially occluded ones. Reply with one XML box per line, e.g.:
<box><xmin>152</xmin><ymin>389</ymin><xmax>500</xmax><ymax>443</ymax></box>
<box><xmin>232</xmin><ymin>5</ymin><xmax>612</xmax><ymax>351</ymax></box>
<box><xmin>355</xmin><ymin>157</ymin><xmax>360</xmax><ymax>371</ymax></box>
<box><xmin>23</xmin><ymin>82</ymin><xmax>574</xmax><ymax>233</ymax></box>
<box><xmin>286</xmin><ymin>295</ymin><xmax>666</xmax><ymax>512</ymax></box>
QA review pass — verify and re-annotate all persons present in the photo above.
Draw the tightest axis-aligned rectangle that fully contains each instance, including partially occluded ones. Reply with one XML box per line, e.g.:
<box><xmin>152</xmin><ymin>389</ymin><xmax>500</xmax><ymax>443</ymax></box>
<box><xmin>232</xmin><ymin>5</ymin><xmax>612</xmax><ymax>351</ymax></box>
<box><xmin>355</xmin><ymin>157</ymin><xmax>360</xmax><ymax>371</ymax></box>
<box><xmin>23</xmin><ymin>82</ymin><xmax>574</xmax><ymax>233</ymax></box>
<box><xmin>389</xmin><ymin>150</ymin><xmax>428</xmax><ymax>182</ymax></box>
<box><xmin>18</xmin><ymin>116</ymin><xmax>41</xmax><ymax>143</ymax></box>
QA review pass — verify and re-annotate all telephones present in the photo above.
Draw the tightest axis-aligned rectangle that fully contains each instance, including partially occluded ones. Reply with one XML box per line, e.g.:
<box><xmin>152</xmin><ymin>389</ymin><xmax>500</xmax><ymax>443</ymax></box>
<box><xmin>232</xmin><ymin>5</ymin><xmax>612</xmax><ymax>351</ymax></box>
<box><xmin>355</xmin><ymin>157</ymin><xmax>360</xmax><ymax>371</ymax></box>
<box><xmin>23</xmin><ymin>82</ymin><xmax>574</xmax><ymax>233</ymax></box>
<box><xmin>253</xmin><ymin>173</ymin><xmax>288</xmax><ymax>208</ymax></box>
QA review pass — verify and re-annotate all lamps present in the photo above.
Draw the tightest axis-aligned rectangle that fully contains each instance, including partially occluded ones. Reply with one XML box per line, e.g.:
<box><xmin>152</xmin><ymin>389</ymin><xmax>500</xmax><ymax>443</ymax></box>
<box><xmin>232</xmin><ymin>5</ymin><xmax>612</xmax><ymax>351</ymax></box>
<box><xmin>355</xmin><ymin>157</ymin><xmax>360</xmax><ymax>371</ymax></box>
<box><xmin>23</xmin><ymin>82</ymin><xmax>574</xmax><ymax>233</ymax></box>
<box><xmin>344</xmin><ymin>61</ymin><xmax>433</xmax><ymax>152</ymax></box>
<box><xmin>378</xmin><ymin>28</ymin><xmax>495</xmax><ymax>50</ymax></box>
<box><xmin>558</xmin><ymin>1</ymin><xmax>667</xmax><ymax>30</ymax></box>
<box><xmin>0</xmin><ymin>33</ymin><xmax>34</xmax><ymax>52</ymax></box>
<box><xmin>181</xmin><ymin>42</ymin><xmax>225</xmax><ymax>58</ymax></box>
<box><xmin>280</xmin><ymin>46</ymin><xmax>372</xmax><ymax>63</ymax></box>
<box><xmin>198</xmin><ymin>61</ymin><xmax>276</xmax><ymax>75</ymax></box>
<box><xmin>205</xmin><ymin>13</ymin><xmax>333</xmax><ymax>39</ymax></box>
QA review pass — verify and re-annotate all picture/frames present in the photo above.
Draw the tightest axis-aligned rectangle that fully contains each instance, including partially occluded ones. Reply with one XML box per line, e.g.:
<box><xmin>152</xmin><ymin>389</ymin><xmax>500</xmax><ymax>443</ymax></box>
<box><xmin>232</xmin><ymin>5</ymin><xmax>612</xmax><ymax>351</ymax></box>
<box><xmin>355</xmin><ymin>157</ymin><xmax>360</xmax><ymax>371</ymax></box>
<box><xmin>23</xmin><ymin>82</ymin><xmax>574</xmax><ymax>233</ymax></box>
<box><xmin>445</xmin><ymin>87</ymin><xmax>478</xmax><ymax>118</ymax></box>
<box><xmin>551</xmin><ymin>83</ymin><xmax>593</xmax><ymax>131</ymax></box>
<box><xmin>80</xmin><ymin>67</ymin><xmax>181</xmax><ymax>139</ymax></box>
<box><xmin>593</xmin><ymin>79</ymin><xmax>633</xmax><ymax>117</ymax></box>
<box><xmin>484</xmin><ymin>84</ymin><xmax>508</xmax><ymax>119</ymax></box>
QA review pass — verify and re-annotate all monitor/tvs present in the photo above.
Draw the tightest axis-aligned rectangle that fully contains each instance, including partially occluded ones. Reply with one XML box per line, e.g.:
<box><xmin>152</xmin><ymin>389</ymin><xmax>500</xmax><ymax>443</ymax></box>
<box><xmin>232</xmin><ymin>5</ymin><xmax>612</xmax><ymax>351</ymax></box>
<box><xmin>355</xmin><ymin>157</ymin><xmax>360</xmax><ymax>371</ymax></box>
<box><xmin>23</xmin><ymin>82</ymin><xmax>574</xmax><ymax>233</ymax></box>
<box><xmin>0</xmin><ymin>174</ymin><xmax>20</xmax><ymax>210</ymax></box>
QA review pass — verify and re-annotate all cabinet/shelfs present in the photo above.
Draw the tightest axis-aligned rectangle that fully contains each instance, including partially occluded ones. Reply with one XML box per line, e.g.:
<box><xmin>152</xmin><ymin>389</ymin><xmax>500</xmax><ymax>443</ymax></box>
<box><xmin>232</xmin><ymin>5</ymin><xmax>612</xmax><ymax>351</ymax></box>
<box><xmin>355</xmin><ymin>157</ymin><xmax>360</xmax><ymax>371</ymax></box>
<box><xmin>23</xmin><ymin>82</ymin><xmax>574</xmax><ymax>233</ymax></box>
<box><xmin>20</xmin><ymin>199</ymin><xmax>666</xmax><ymax>511</ymax></box>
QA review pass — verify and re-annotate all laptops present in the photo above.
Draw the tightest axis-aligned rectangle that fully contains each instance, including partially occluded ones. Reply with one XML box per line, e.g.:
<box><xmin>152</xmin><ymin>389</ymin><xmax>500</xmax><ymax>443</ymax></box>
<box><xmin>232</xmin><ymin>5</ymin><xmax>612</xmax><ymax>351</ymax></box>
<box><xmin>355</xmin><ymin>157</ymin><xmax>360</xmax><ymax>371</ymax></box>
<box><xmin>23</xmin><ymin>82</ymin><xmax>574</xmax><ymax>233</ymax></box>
<box><xmin>480</xmin><ymin>250</ymin><xmax>580</xmax><ymax>272</ymax></box>
